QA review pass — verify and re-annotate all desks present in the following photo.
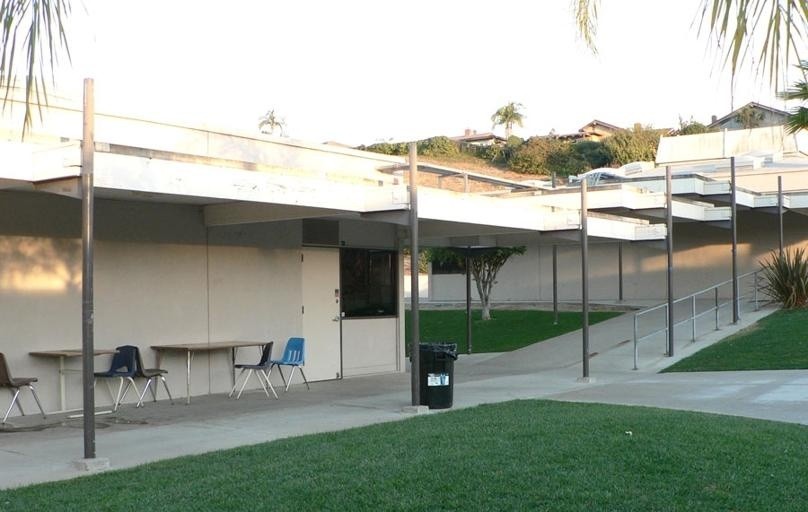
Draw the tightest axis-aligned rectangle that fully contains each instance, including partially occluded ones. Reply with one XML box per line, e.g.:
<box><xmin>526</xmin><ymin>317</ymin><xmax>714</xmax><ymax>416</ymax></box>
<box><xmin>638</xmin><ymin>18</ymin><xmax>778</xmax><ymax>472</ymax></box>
<box><xmin>29</xmin><ymin>349</ymin><xmax>120</xmax><ymax>419</ymax></box>
<box><xmin>148</xmin><ymin>341</ymin><xmax>275</xmax><ymax>404</ymax></box>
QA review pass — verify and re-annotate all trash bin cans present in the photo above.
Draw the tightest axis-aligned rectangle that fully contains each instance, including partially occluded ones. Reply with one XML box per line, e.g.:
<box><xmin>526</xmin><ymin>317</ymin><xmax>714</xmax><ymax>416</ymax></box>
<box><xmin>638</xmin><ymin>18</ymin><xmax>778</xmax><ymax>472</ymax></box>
<box><xmin>407</xmin><ymin>341</ymin><xmax>459</xmax><ymax>409</ymax></box>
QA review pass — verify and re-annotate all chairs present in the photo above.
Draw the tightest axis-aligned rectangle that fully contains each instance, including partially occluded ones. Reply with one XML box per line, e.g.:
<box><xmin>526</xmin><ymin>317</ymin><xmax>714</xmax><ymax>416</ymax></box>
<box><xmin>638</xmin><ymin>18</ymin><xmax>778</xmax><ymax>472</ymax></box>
<box><xmin>129</xmin><ymin>344</ymin><xmax>174</xmax><ymax>410</ymax></box>
<box><xmin>0</xmin><ymin>352</ymin><xmax>47</xmax><ymax>423</ymax></box>
<box><xmin>93</xmin><ymin>346</ymin><xmax>144</xmax><ymax>411</ymax></box>
<box><xmin>266</xmin><ymin>337</ymin><xmax>309</xmax><ymax>393</ymax></box>
<box><xmin>229</xmin><ymin>341</ymin><xmax>277</xmax><ymax>401</ymax></box>
